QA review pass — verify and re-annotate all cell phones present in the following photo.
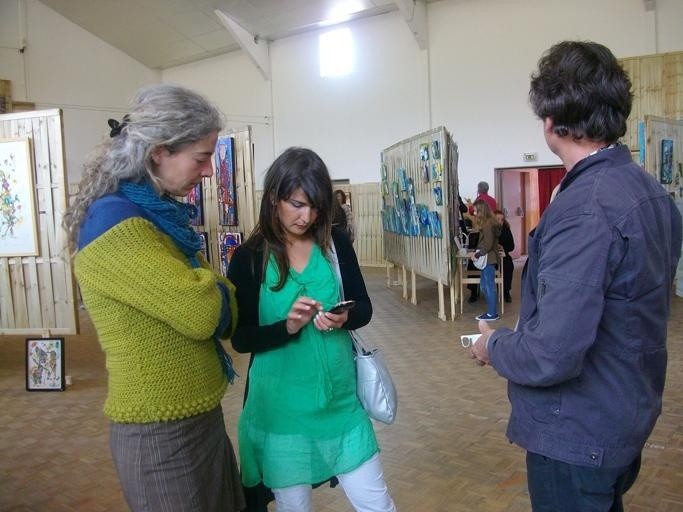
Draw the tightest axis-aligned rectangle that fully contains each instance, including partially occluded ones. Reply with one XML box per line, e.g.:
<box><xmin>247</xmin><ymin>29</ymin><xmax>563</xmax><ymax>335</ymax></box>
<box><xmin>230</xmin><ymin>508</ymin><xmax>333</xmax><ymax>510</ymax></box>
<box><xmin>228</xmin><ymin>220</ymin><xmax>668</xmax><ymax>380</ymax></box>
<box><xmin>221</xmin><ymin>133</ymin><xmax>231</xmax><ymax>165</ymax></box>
<box><xmin>330</xmin><ymin>298</ymin><xmax>353</xmax><ymax>315</ymax></box>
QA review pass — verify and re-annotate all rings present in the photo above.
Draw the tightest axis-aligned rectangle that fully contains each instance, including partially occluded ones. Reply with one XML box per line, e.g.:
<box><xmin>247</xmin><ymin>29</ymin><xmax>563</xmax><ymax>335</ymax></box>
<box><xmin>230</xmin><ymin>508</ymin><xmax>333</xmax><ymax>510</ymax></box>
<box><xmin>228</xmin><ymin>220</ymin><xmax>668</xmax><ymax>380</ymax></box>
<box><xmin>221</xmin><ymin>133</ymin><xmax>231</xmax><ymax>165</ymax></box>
<box><xmin>326</xmin><ymin>327</ymin><xmax>333</xmax><ymax>332</ymax></box>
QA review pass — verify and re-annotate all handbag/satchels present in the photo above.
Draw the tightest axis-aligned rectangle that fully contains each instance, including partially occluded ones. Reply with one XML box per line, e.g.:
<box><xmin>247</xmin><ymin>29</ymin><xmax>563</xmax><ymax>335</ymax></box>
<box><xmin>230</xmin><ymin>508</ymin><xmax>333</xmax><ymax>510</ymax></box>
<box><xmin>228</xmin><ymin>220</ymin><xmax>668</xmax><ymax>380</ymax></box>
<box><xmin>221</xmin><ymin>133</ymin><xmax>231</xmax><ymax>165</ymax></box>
<box><xmin>473</xmin><ymin>250</ymin><xmax>488</xmax><ymax>270</ymax></box>
<box><xmin>325</xmin><ymin>231</ymin><xmax>396</xmax><ymax>425</ymax></box>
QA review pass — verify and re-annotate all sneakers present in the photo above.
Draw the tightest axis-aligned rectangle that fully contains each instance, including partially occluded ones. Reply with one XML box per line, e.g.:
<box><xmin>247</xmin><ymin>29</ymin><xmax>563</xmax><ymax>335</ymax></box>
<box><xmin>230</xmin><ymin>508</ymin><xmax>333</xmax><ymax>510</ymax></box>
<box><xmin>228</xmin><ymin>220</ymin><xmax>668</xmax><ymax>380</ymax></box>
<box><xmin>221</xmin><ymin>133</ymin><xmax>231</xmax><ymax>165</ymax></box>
<box><xmin>475</xmin><ymin>313</ymin><xmax>499</xmax><ymax>320</ymax></box>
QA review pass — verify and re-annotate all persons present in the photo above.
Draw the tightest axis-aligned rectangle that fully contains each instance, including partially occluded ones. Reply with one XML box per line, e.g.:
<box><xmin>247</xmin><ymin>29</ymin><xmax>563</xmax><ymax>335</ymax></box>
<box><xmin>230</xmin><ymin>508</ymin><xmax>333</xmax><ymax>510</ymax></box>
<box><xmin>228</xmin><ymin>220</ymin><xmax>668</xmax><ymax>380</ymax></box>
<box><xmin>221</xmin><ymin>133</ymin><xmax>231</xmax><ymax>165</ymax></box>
<box><xmin>493</xmin><ymin>211</ymin><xmax>515</xmax><ymax>302</ymax></box>
<box><xmin>467</xmin><ymin>42</ymin><xmax>682</xmax><ymax>511</ymax></box>
<box><xmin>471</xmin><ymin>201</ymin><xmax>499</xmax><ymax>321</ymax></box>
<box><xmin>228</xmin><ymin>147</ymin><xmax>396</xmax><ymax>511</ymax></box>
<box><xmin>467</xmin><ymin>183</ymin><xmax>498</xmax><ymax>231</ymax></box>
<box><xmin>70</xmin><ymin>86</ymin><xmax>243</xmax><ymax>508</ymax></box>
<box><xmin>333</xmin><ymin>190</ymin><xmax>355</xmax><ymax>243</ymax></box>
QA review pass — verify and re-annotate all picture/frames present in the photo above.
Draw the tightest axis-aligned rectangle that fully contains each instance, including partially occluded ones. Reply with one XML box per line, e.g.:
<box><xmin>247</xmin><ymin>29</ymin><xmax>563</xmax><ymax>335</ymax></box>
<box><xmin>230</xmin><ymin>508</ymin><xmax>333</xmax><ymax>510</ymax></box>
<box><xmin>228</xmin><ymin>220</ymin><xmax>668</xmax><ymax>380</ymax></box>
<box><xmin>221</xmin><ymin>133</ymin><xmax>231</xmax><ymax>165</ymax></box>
<box><xmin>24</xmin><ymin>338</ymin><xmax>66</xmax><ymax>391</ymax></box>
<box><xmin>214</xmin><ymin>137</ymin><xmax>238</xmax><ymax>227</ymax></box>
<box><xmin>187</xmin><ymin>183</ymin><xmax>204</xmax><ymax>226</ymax></box>
<box><xmin>661</xmin><ymin>140</ymin><xmax>673</xmax><ymax>183</ymax></box>
<box><xmin>198</xmin><ymin>233</ymin><xmax>208</xmax><ymax>262</ymax></box>
<box><xmin>217</xmin><ymin>232</ymin><xmax>243</xmax><ymax>277</ymax></box>
<box><xmin>0</xmin><ymin>136</ymin><xmax>39</xmax><ymax>257</ymax></box>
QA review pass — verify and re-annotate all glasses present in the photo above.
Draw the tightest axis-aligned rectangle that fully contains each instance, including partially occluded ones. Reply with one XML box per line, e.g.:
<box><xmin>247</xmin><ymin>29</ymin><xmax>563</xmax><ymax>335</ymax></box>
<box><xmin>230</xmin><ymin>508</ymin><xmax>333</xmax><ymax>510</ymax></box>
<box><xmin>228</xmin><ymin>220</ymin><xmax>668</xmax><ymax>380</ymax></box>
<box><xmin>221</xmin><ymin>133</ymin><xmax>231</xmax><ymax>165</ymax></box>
<box><xmin>460</xmin><ymin>334</ymin><xmax>485</xmax><ymax>349</ymax></box>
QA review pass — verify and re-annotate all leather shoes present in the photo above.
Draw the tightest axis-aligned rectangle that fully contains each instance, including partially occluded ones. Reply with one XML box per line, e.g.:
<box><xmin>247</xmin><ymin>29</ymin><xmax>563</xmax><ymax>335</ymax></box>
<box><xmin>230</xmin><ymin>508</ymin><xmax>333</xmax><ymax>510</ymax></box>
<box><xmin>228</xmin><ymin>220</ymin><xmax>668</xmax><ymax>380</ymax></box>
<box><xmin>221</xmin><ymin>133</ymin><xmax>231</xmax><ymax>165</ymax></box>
<box><xmin>506</xmin><ymin>293</ymin><xmax>510</xmax><ymax>302</ymax></box>
<box><xmin>469</xmin><ymin>297</ymin><xmax>476</xmax><ymax>303</ymax></box>
